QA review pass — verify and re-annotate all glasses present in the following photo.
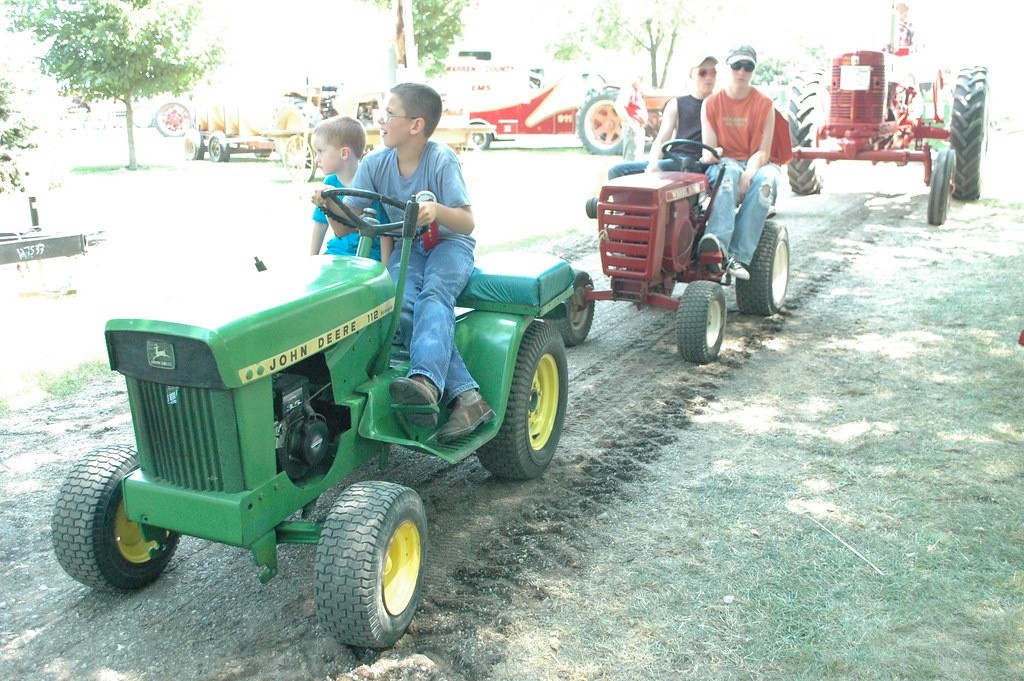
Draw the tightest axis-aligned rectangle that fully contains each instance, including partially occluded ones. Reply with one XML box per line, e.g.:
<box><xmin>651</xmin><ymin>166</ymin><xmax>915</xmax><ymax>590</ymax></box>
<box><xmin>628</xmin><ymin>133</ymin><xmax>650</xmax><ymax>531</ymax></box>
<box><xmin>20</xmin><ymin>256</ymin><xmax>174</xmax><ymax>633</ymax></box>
<box><xmin>382</xmin><ymin>111</ymin><xmax>417</xmax><ymax>123</ymax></box>
<box><xmin>698</xmin><ymin>70</ymin><xmax>717</xmax><ymax>77</ymax></box>
<box><xmin>731</xmin><ymin>61</ymin><xmax>755</xmax><ymax>72</ymax></box>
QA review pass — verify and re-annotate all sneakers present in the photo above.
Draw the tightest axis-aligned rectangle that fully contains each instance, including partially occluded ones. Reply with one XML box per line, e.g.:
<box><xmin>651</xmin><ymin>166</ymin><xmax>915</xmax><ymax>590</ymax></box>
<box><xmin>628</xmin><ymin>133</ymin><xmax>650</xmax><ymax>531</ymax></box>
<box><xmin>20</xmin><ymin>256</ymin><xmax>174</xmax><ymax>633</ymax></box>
<box><xmin>726</xmin><ymin>259</ymin><xmax>751</xmax><ymax>281</ymax></box>
<box><xmin>697</xmin><ymin>232</ymin><xmax>724</xmax><ymax>273</ymax></box>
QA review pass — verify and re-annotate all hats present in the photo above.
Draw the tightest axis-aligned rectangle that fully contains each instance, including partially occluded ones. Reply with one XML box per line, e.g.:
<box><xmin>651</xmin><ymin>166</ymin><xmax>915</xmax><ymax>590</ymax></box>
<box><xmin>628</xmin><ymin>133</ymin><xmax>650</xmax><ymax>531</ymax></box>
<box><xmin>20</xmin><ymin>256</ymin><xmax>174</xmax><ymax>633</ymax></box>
<box><xmin>689</xmin><ymin>54</ymin><xmax>718</xmax><ymax>73</ymax></box>
<box><xmin>725</xmin><ymin>45</ymin><xmax>757</xmax><ymax>65</ymax></box>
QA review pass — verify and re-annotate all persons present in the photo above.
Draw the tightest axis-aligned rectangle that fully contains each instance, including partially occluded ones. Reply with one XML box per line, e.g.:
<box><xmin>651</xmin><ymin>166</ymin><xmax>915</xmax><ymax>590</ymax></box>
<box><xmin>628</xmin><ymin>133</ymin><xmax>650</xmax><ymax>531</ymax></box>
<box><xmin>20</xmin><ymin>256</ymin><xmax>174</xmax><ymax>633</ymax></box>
<box><xmin>692</xmin><ymin>43</ymin><xmax>783</xmax><ymax>284</ymax></box>
<box><xmin>310</xmin><ymin>81</ymin><xmax>495</xmax><ymax>445</ymax></box>
<box><xmin>307</xmin><ymin>117</ymin><xmax>392</xmax><ymax>272</ymax></box>
<box><xmin>614</xmin><ymin>67</ymin><xmax>647</xmax><ymax>164</ymax></box>
<box><xmin>605</xmin><ymin>50</ymin><xmax>719</xmax><ymax>206</ymax></box>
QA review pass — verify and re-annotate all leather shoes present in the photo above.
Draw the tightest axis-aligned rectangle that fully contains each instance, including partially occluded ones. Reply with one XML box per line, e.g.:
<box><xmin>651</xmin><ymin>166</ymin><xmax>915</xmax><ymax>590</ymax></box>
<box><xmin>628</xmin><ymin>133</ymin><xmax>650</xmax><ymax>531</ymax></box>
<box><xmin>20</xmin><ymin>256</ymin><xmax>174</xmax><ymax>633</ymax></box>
<box><xmin>389</xmin><ymin>376</ymin><xmax>439</xmax><ymax>429</ymax></box>
<box><xmin>437</xmin><ymin>398</ymin><xmax>497</xmax><ymax>443</ymax></box>
